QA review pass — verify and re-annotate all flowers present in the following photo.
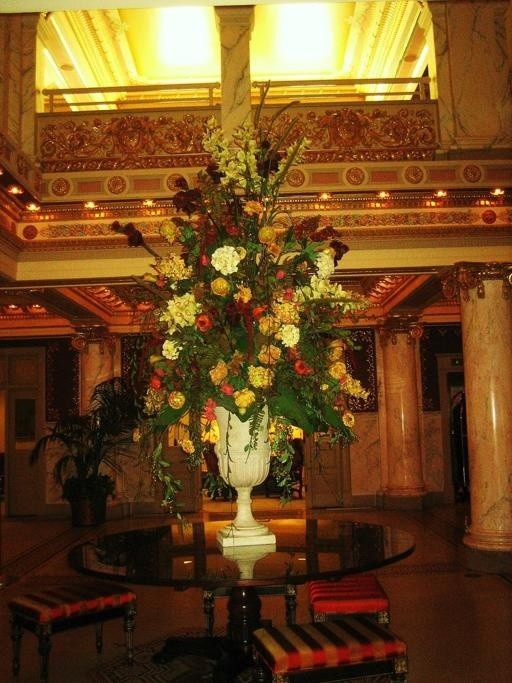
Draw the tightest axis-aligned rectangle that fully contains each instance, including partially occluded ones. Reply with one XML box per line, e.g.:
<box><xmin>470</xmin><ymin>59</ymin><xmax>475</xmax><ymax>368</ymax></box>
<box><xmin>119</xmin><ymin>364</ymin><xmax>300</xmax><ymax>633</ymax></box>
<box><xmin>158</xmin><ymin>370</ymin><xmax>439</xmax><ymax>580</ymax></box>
<box><xmin>109</xmin><ymin>79</ymin><xmax>372</xmax><ymax>532</ymax></box>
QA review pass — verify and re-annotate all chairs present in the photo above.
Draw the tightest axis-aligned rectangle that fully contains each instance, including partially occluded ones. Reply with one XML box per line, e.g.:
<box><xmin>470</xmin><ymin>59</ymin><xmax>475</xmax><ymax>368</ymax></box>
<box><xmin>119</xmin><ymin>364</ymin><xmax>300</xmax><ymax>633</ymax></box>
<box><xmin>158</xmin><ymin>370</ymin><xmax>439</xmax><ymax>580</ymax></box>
<box><xmin>202</xmin><ymin>435</ymin><xmax>304</xmax><ymax>498</ymax></box>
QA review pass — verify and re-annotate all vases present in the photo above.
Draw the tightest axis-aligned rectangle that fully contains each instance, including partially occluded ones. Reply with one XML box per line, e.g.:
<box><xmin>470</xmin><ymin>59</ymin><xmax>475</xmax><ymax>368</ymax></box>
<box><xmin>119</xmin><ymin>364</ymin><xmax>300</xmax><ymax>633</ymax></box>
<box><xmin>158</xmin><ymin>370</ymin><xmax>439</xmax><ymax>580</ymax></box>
<box><xmin>213</xmin><ymin>404</ymin><xmax>276</xmax><ymax>548</ymax></box>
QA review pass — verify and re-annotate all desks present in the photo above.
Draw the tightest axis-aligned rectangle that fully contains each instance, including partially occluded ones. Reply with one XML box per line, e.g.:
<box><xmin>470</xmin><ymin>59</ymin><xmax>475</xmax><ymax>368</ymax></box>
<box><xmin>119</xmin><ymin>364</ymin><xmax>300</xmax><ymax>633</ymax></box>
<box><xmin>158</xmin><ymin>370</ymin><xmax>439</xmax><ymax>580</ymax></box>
<box><xmin>68</xmin><ymin>517</ymin><xmax>416</xmax><ymax>682</ymax></box>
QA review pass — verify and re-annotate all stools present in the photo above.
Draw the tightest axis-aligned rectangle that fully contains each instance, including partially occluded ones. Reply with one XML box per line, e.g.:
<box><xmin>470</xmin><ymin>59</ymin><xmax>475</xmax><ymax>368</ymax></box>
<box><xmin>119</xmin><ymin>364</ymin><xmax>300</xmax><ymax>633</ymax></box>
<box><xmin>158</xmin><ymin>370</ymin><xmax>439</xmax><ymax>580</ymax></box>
<box><xmin>8</xmin><ymin>580</ymin><xmax>137</xmax><ymax>683</ymax></box>
<box><xmin>309</xmin><ymin>575</ymin><xmax>391</xmax><ymax>628</ymax></box>
<box><xmin>251</xmin><ymin>616</ymin><xmax>408</xmax><ymax>683</ymax></box>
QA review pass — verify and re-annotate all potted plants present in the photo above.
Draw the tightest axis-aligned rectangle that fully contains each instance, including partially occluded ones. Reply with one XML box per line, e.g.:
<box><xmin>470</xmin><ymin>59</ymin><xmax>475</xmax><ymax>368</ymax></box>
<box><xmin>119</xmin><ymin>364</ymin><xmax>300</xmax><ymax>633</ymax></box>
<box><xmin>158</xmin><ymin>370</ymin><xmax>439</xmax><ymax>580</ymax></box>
<box><xmin>28</xmin><ymin>414</ymin><xmax>141</xmax><ymax>527</ymax></box>
